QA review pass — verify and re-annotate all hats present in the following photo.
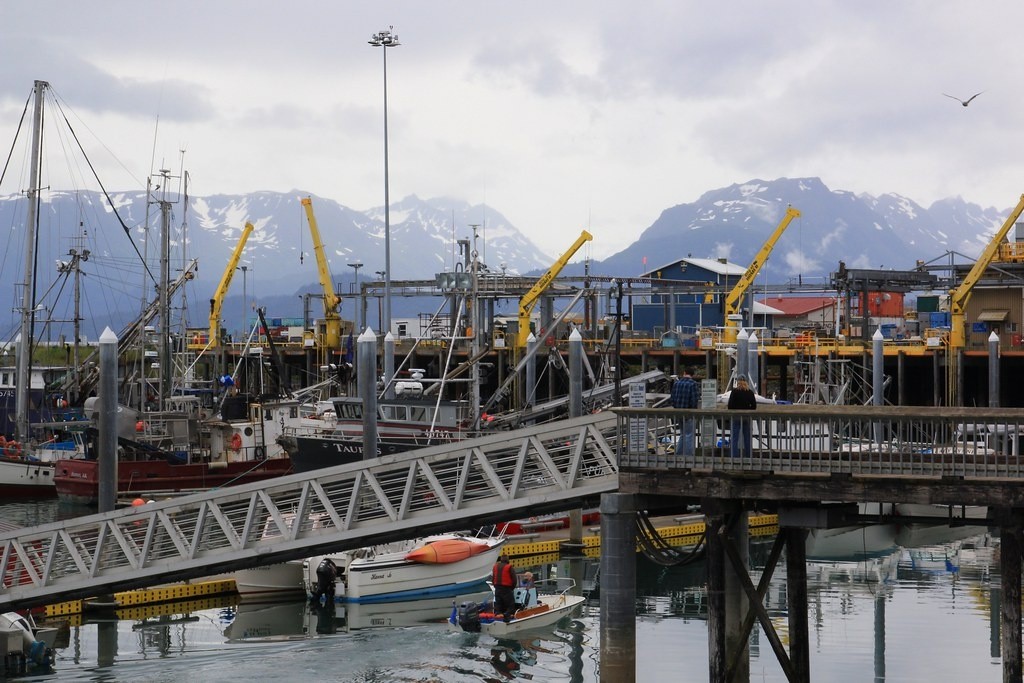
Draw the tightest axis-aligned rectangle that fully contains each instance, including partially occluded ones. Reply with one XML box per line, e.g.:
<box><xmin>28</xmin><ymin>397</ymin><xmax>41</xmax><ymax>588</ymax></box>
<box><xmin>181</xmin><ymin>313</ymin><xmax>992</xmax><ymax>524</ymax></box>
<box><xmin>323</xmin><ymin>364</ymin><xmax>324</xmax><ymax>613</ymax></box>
<box><xmin>501</xmin><ymin>555</ymin><xmax>509</xmax><ymax>563</ymax></box>
<box><xmin>735</xmin><ymin>374</ymin><xmax>747</xmax><ymax>381</ymax></box>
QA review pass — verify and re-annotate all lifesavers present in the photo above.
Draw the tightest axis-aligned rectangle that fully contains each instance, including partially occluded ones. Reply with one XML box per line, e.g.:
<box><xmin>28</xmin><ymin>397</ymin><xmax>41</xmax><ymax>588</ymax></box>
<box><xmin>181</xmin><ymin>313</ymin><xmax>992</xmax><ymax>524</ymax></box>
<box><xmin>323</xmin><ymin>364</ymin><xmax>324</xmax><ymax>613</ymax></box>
<box><xmin>231</xmin><ymin>434</ymin><xmax>241</xmax><ymax>450</ymax></box>
<box><xmin>4</xmin><ymin>441</ymin><xmax>21</xmax><ymax>458</ymax></box>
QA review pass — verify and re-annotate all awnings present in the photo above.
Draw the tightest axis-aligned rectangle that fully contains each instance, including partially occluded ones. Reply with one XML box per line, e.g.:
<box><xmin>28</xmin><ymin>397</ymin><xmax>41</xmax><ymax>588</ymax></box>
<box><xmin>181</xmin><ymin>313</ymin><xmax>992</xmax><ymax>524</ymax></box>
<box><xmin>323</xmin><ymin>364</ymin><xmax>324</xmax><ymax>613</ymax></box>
<box><xmin>976</xmin><ymin>310</ymin><xmax>1009</xmax><ymax>321</ymax></box>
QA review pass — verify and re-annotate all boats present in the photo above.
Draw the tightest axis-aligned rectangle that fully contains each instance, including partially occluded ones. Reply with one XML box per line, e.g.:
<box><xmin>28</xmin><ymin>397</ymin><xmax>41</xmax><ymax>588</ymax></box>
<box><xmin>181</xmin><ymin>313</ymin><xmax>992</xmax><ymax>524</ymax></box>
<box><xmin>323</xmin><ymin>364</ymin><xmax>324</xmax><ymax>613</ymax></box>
<box><xmin>893</xmin><ymin>518</ymin><xmax>990</xmax><ymax>614</ymax></box>
<box><xmin>447</xmin><ymin>573</ymin><xmax>585</xmax><ymax>635</ymax></box>
<box><xmin>0</xmin><ymin>611</ymin><xmax>58</xmax><ymax>670</ymax></box>
<box><xmin>781</xmin><ymin>519</ymin><xmax>902</xmax><ymax>654</ymax></box>
<box><xmin>0</xmin><ymin>76</ymin><xmax>1024</xmax><ymax>601</ymax></box>
<box><xmin>222</xmin><ymin>593</ymin><xmax>307</xmax><ymax>645</ymax></box>
<box><xmin>302</xmin><ymin>582</ymin><xmax>493</xmax><ymax>640</ymax></box>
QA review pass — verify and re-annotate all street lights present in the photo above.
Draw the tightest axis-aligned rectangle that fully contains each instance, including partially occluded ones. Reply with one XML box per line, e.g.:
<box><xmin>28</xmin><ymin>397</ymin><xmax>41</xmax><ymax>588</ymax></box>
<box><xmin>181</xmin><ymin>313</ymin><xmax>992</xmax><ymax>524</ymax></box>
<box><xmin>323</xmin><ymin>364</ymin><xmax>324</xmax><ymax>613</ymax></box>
<box><xmin>347</xmin><ymin>263</ymin><xmax>363</xmax><ymax>334</ymax></box>
<box><xmin>236</xmin><ymin>266</ymin><xmax>253</xmax><ymax>341</ymax></box>
<box><xmin>375</xmin><ymin>271</ymin><xmax>386</xmax><ymax>332</ymax></box>
<box><xmin>366</xmin><ymin>25</ymin><xmax>401</xmax><ymax>332</ymax></box>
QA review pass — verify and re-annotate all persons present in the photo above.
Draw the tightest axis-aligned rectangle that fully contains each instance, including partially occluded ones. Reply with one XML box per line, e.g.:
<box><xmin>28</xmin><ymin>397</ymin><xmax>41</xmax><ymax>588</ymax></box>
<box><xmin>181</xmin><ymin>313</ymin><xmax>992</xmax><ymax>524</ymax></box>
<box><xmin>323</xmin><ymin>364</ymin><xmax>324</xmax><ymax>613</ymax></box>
<box><xmin>727</xmin><ymin>373</ymin><xmax>758</xmax><ymax>459</ymax></box>
<box><xmin>521</xmin><ymin>572</ymin><xmax>542</xmax><ymax>604</ymax></box>
<box><xmin>492</xmin><ymin>554</ymin><xmax>518</xmax><ymax>623</ymax></box>
<box><xmin>670</xmin><ymin>364</ymin><xmax>699</xmax><ymax>456</ymax></box>
